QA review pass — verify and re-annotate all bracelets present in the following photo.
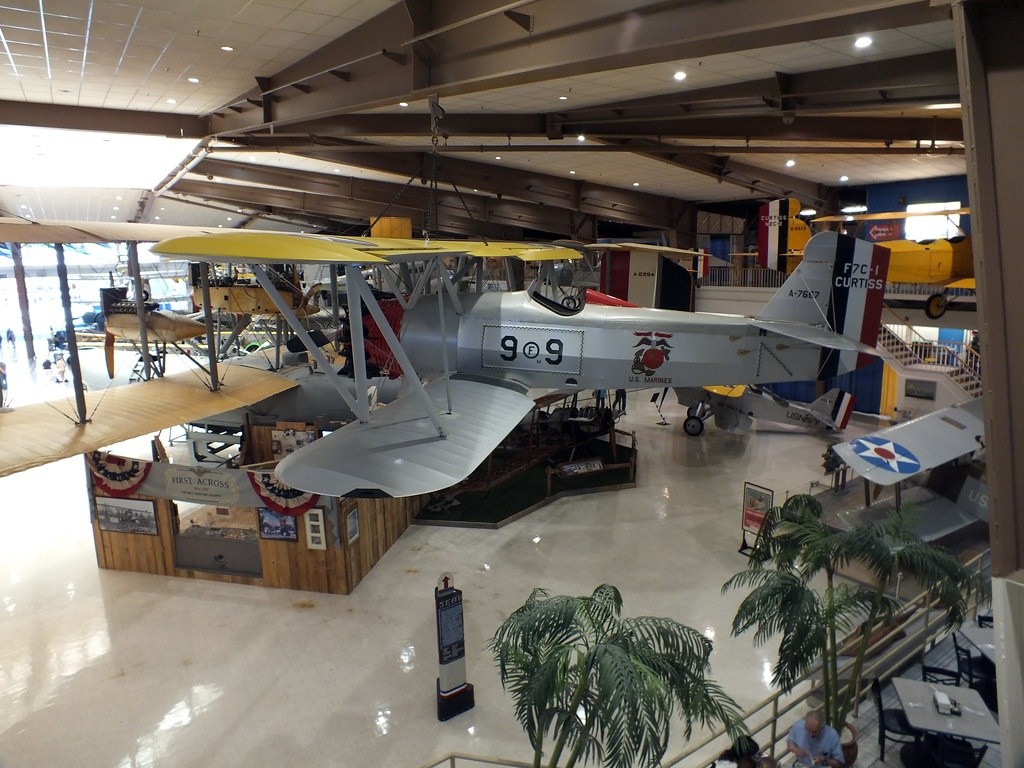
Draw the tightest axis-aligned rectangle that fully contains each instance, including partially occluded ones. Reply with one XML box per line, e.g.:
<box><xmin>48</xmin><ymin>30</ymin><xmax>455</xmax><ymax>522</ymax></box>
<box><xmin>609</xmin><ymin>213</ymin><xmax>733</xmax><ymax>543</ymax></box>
<box><xmin>822</xmin><ymin>757</ymin><xmax>827</xmax><ymax>764</ymax></box>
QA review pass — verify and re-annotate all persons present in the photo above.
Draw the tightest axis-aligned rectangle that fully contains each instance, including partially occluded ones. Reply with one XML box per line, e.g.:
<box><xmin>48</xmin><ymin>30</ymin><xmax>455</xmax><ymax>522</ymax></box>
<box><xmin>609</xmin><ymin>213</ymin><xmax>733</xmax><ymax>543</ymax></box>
<box><xmin>709</xmin><ymin>735</ymin><xmax>759</xmax><ymax>768</ymax></box>
<box><xmin>0</xmin><ymin>327</ymin><xmax>76</xmax><ymax>408</ymax></box>
<box><xmin>786</xmin><ymin>709</ymin><xmax>846</xmax><ymax>768</ymax></box>
<box><xmin>612</xmin><ymin>389</ymin><xmax>628</xmax><ymax>416</ymax></box>
<box><xmin>594</xmin><ymin>389</ymin><xmax>608</xmax><ymax>409</ymax></box>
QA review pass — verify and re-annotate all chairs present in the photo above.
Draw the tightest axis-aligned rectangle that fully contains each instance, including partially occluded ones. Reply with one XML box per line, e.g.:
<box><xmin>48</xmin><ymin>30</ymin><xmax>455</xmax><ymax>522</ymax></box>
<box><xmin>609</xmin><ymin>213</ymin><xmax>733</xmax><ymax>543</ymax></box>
<box><xmin>870</xmin><ymin>614</ymin><xmax>999</xmax><ymax>768</ymax></box>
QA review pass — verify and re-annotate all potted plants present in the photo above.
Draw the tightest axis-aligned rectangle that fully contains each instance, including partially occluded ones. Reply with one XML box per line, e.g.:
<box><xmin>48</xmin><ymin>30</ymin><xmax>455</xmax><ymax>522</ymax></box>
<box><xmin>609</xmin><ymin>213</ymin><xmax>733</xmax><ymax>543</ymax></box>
<box><xmin>719</xmin><ymin>491</ymin><xmax>992</xmax><ymax>768</ymax></box>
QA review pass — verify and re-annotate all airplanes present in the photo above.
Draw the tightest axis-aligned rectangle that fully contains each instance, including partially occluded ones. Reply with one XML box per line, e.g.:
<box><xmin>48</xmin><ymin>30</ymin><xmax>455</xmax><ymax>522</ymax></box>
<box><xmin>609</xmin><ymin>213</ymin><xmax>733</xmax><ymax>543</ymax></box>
<box><xmin>669</xmin><ymin>384</ymin><xmax>856</xmax><ymax>437</ymax></box>
<box><xmin>0</xmin><ymin>217</ymin><xmax>399</xmax><ymax>479</ymax></box>
<box><xmin>809</xmin><ymin>207</ymin><xmax>977</xmax><ymax>322</ymax></box>
<box><xmin>831</xmin><ymin>396</ymin><xmax>986</xmax><ymax>486</ymax></box>
<box><xmin>148</xmin><ymin>224</ymin><xmax>893</xmax><ymax>501</ymax></box>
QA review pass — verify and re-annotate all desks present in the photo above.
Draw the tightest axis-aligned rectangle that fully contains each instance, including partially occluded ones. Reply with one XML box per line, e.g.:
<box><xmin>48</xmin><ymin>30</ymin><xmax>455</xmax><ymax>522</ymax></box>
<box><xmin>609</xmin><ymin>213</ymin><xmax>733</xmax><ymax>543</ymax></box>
<box><xmin>958</xmin><ymin>628</ymin><xmax>995</xmax><ymax>665</ymax></box>
<box><xmin>892</xmin><ymin>677</ymin><xmax>999</xmax><ymax>743</ymax></box>
